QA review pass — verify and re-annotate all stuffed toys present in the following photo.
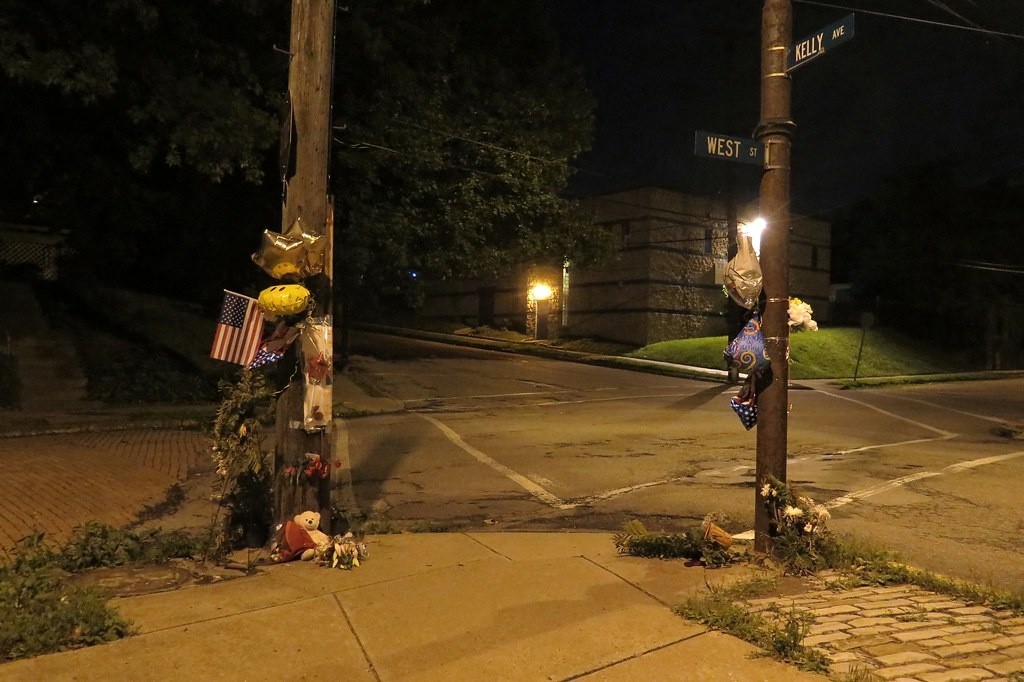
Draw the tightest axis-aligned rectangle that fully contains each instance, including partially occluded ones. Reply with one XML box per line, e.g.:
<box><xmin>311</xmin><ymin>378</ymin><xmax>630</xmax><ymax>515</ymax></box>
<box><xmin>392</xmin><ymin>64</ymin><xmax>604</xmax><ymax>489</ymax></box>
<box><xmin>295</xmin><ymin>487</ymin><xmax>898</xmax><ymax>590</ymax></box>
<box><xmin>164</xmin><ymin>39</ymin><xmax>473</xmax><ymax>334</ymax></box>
<box><xmin>294</xmin><ymin>511</ymin><xmax>331</xmax><ymax>561</ymax></box>
<box><xmin>788</xmin><ymin>302</ymin><xmax>819</xmax><ymax>332</ymax></box>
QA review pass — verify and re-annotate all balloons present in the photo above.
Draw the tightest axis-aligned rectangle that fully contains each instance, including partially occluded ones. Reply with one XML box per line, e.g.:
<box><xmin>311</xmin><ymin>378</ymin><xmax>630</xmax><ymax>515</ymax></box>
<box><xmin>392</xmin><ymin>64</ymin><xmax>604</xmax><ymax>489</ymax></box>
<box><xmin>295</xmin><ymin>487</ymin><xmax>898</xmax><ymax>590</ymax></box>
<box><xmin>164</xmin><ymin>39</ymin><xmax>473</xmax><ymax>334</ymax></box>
<box><xmin>251</xmin><ymin>217</ymin><xmax>327</xmax><ymax>316</ymax></box>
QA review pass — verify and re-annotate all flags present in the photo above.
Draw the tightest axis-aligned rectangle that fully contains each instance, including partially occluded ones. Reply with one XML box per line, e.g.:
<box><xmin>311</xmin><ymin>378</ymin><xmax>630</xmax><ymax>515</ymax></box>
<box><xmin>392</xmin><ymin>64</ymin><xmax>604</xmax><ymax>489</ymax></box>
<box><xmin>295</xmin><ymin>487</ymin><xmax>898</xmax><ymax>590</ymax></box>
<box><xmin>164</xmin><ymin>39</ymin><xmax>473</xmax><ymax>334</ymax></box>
<box><xmin>210</xmin><ymin>289</ymin><xmax>266</xmax><ymax>367</ymax></box>
